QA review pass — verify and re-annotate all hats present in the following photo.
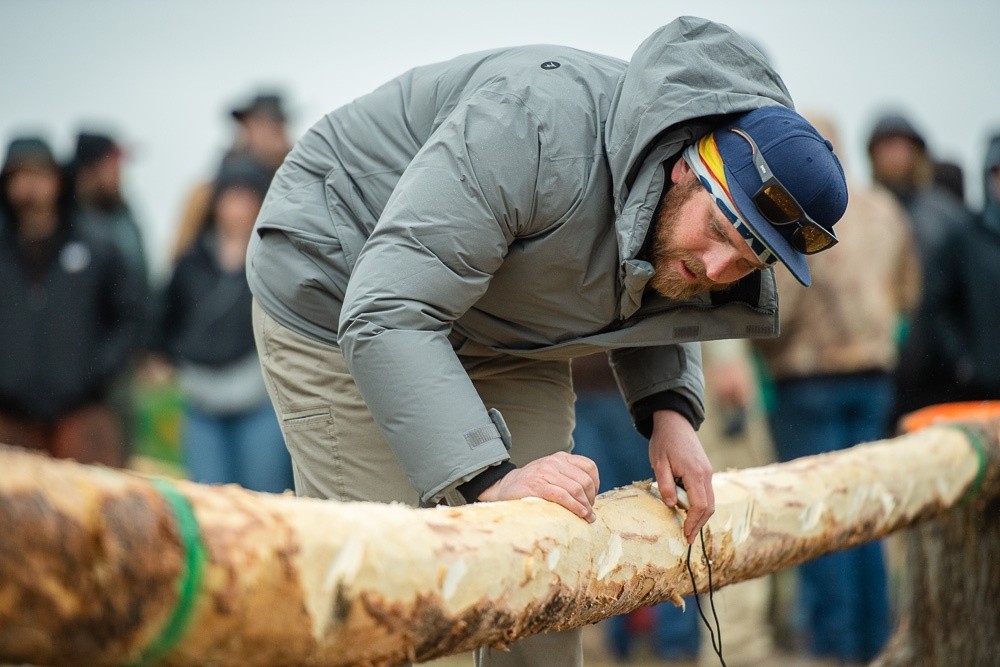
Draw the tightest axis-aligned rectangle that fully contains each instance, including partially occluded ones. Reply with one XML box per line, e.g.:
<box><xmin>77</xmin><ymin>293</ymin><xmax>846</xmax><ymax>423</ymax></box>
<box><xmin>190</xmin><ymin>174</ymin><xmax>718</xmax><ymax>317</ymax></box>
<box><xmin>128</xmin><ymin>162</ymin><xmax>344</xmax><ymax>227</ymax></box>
<box><xmin>716</xmin><ymin>104</ymin><xmax>848</xmax><ymax>287</ymax></box>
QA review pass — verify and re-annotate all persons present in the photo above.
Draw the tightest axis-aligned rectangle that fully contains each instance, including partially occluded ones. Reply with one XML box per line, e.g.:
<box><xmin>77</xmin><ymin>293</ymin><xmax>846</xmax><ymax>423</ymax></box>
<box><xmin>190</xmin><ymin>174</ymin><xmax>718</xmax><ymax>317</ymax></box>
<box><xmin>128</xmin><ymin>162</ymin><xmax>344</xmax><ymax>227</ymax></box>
<box><xmin>744</xmin><ymin>114</ymin><xmax>1000</xmax><ymax>667</ymax></box>
<box><xmin>0</xmin><ymin>96</ymin><xmax>292</xmax><ymax>494</ymax></box>
<box><xmin>573</xmin><ymin>348</ymin><xmax>711</xmax><ymax>667</ymax></box>
<box><xmin>246</xmin><ymin>16</ymin><xmax>849</xmax><ymax>667</ymax></box>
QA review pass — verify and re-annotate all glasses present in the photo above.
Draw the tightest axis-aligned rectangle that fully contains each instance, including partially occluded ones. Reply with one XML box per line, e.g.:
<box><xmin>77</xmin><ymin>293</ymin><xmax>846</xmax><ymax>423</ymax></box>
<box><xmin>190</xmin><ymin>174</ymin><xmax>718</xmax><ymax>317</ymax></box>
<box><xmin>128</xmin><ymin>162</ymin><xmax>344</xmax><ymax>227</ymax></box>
<box><xmin>727</xmin><ymin>125</ymin><xmax>839</xmax><ymax>254</ymax></box>
